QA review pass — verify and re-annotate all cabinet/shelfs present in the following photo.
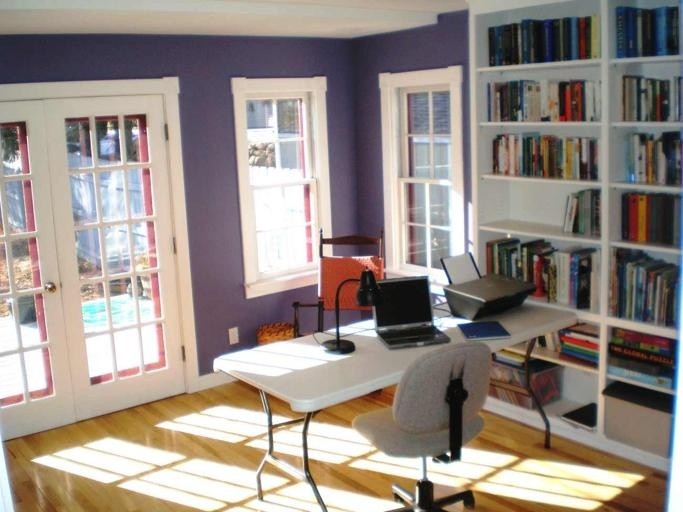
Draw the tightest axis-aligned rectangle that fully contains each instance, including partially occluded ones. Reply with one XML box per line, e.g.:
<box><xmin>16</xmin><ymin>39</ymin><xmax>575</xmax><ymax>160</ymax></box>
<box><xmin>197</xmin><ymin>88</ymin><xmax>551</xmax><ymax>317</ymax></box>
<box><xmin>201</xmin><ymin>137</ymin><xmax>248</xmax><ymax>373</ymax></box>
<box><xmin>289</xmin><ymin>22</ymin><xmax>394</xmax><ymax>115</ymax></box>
<box><xmin>470</xmin><ymin>2</ymin><xmax>683</xmax><ymax>475</ymax></box>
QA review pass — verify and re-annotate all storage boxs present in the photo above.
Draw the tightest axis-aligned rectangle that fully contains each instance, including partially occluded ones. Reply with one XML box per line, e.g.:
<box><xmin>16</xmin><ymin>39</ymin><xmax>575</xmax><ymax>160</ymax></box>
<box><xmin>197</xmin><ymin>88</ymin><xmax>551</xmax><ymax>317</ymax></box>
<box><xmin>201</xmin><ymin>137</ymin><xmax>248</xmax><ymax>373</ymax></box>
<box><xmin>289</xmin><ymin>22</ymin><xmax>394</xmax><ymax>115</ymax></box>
<box><xmin>598</xmin><ymin>381</ymin><xmax>675</xmax><ymax>460</ymax></box>
<box><xmin>488</xmin><ymin>354</ymin><xmax>566</xmax><ymax>411</ymax></box>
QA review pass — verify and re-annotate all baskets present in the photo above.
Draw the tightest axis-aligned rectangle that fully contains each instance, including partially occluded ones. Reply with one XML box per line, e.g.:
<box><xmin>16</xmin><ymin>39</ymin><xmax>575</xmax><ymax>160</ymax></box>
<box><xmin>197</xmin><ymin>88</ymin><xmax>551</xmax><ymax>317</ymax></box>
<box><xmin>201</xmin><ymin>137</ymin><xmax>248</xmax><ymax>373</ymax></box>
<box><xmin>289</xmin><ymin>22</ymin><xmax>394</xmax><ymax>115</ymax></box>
<box><xmin>256</xmin><ymin>321</ymin><xmax>296</xmax><ymax>346</ymax></box>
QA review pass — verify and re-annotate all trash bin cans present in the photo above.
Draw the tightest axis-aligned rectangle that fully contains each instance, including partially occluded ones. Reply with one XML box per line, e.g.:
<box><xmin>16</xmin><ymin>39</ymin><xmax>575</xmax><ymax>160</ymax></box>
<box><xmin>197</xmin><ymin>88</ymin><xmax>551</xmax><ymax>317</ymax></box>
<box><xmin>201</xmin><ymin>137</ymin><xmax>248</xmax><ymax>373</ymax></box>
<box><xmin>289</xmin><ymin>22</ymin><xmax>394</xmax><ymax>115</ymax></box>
<box><xmin>7</xmin><ymin>289</ymin><xmax>35</xmax><ymax>323</ymax></box>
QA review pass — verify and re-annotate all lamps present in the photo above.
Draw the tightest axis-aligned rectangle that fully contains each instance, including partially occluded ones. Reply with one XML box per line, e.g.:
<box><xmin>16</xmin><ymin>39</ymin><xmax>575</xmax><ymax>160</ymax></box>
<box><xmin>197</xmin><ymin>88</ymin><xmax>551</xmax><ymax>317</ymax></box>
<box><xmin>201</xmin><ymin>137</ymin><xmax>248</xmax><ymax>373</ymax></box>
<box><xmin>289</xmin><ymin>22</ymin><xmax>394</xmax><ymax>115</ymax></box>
<box><xmin>321</xmin><ymin>266</ymin><xmax>380</xmax><ymax>355</ymax></box>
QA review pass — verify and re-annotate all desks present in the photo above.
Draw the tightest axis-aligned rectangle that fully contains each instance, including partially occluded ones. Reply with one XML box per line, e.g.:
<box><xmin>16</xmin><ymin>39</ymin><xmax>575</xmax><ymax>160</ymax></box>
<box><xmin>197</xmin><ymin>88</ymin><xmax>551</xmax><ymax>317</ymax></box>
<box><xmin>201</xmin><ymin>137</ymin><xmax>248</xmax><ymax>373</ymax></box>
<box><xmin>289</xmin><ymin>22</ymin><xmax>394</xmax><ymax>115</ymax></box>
<box><xmin>210</xmin><ymin>300</ymin><xmax>579</xmax><ymax>512</ymax></box>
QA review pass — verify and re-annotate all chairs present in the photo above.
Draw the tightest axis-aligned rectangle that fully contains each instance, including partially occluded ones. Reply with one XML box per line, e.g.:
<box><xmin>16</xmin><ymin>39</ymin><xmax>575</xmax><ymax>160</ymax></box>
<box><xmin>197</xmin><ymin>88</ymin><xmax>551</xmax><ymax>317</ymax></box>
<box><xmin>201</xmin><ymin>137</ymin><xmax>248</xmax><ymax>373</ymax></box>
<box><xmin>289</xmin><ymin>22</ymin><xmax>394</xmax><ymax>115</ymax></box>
<box><xmin>351</xmin><ymin>341</ymin><xmax>492</xmax><ymax>512</ymax></box>
<box><xmin>291</xmin><ymin>225</ymin><xmax>388</xmax><ymax>336</ymax></box>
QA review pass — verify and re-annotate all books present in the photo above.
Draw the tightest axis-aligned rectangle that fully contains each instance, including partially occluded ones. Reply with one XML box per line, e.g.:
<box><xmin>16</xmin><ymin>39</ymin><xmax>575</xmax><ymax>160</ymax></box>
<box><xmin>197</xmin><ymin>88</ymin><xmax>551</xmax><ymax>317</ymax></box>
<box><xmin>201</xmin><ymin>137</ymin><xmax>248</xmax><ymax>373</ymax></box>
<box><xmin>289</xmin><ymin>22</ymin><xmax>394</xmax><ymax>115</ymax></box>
<box><xmin>487</xmin><ymin>237</ymin><xmax>677</xmax><ymax>396</ymax></box>
<box><xmin>491</xmin><ymin>130</ymin><xmax>682</xmax><ymax>249</ymax></box>
<box><xmin>487</xmin><ymin>5</ymin><xmax>680</xmax><ymax>123</ymax></box>
<box><xmin>456</xmin><ymin>320</ymin><xmax>512</xmax><ymax>341</ymax></box>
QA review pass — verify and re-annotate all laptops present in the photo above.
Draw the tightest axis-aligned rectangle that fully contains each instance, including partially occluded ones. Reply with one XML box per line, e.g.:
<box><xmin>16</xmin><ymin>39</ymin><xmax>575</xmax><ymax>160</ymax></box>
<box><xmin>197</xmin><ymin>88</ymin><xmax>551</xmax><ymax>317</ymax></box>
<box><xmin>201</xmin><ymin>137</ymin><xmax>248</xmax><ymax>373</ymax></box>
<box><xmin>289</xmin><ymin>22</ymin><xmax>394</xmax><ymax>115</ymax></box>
<box><xmin>372</xmin><ymin>276</ymin><xmax>450</xmax><ymax>349</ymax></box>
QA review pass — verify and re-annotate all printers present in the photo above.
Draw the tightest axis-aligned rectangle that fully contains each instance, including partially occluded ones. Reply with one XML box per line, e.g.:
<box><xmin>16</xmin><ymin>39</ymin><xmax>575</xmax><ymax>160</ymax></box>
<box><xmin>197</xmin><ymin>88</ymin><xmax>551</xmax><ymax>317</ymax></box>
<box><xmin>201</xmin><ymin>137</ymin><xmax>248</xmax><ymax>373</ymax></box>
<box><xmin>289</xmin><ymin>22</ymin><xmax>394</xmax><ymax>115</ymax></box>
<box><xmin>440</xmin><ymin>252</ymin><xmax>537</xmax><ymax>321</ymax></box>
